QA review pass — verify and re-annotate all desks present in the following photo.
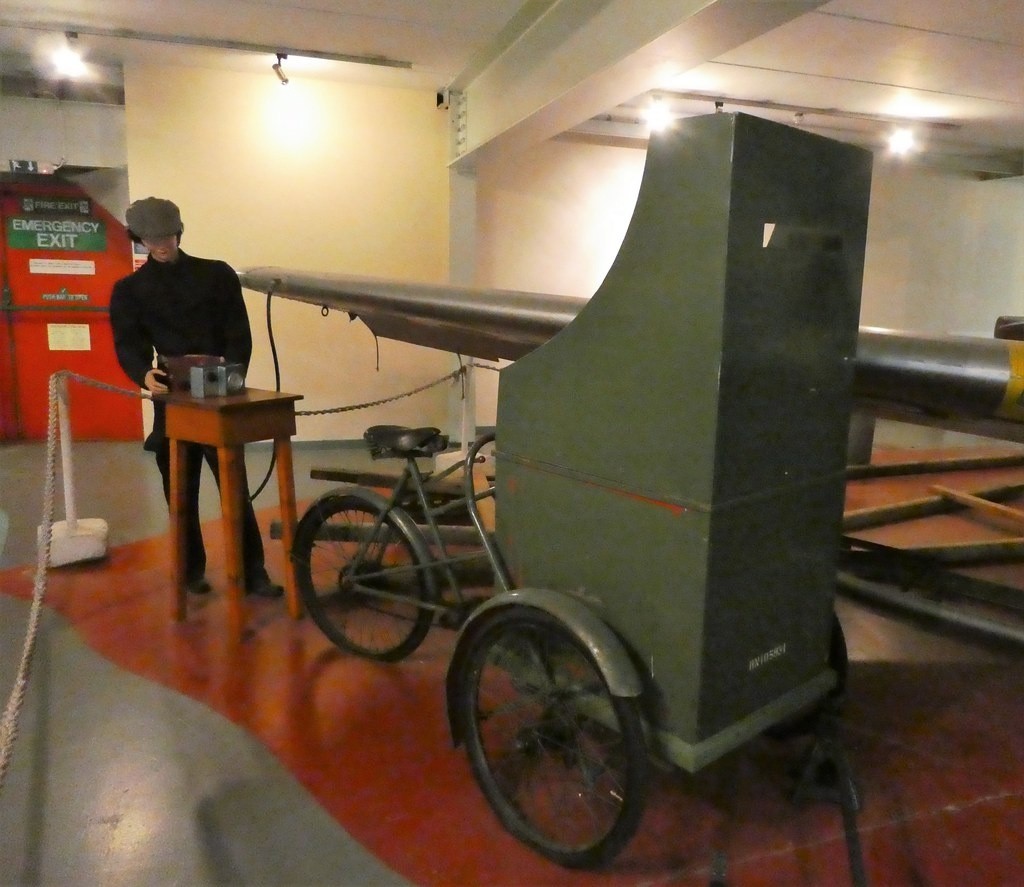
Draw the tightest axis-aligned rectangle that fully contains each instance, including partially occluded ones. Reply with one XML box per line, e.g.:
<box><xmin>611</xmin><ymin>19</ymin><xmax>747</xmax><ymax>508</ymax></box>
<box><xmin>165</xmin><ymin>386</ymin><xmax>304</xmax><ymax>643</ymax></box>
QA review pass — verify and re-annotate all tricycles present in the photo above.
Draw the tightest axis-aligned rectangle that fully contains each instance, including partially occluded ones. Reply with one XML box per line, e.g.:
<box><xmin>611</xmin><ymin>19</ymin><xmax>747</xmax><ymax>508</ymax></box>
<box><xmin>289</xmin><ymin>109</ymin><xmax>875</xmax><ymax>870</ymax></box>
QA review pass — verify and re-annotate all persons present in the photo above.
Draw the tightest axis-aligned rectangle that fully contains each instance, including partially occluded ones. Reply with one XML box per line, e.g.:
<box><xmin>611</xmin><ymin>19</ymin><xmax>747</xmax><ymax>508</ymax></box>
<box><xmin>109</xmin><ymin>197</ymin><xmax>286</xmax><ymax>596</ymax></box>
